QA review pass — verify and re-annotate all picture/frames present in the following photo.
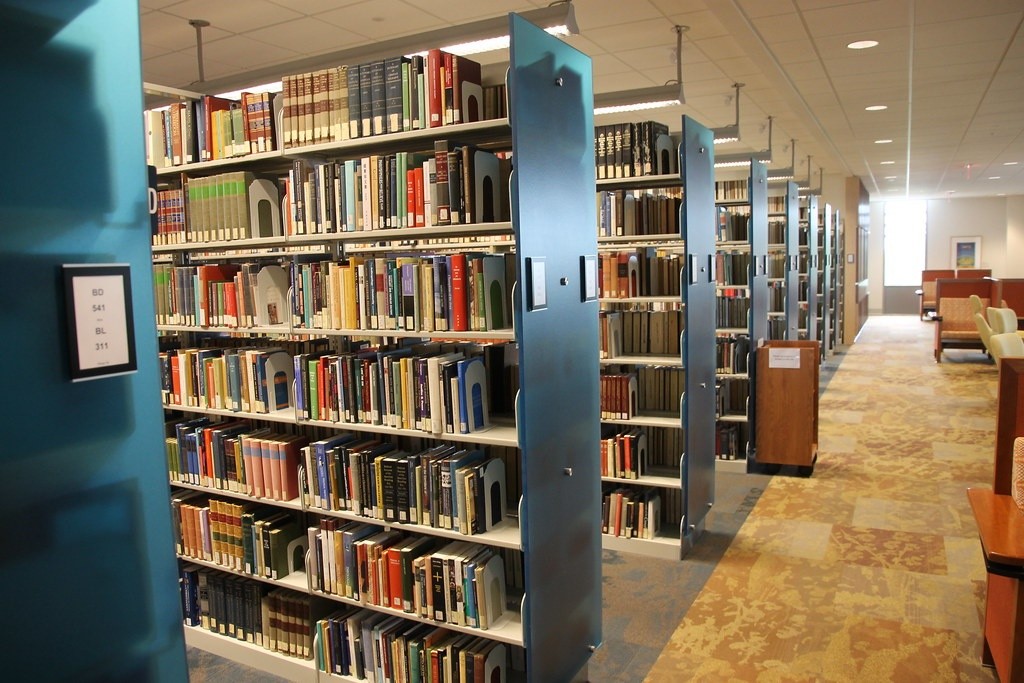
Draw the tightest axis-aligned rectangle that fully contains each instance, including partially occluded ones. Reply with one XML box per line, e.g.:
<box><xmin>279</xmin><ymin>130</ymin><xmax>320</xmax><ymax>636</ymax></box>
<box><xmin>949</xmin><ymin>235</ymin><xmax>983</xmax><ymax>269</ymax></box>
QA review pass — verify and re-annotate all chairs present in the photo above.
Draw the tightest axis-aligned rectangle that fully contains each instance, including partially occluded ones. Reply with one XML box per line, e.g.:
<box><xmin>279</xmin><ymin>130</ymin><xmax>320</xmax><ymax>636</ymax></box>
<box><xmin>922</xmin><ymin>266</ymin><xmax>1024</xmax><ymax>683</ymax></box>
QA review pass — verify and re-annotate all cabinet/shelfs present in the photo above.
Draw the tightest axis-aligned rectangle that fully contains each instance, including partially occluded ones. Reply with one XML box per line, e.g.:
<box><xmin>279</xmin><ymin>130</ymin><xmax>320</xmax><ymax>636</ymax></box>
<box><xmin>0</xmin><ymin>0</ymin><xmax>844</xmax><ymax>683</ymax></box>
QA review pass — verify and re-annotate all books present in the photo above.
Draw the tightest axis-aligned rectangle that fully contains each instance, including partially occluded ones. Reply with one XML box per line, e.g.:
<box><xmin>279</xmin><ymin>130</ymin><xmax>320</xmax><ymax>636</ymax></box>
<box><xmin>143</xmin><ymin>47</ymin><xmax>521</xmax><ymax>683</ymax></box>
<box><xmin>595</xmin><ymin>120</ymin><xmax>683</xmax><ymax>542</ymax></box>
<box><xmin>767</xmin><ymin>196</ymin><xmax>787</xmax><ymax>342</ymax></box>
<box><xmin>714</xmin><ymin>178</ymin><xmax>750</xmax><ymax>460</ymax></box>
<box><xmin>798</xmin><ymin>205</ymin><xmax>843</xmax><ymax>350</ymax></box>
